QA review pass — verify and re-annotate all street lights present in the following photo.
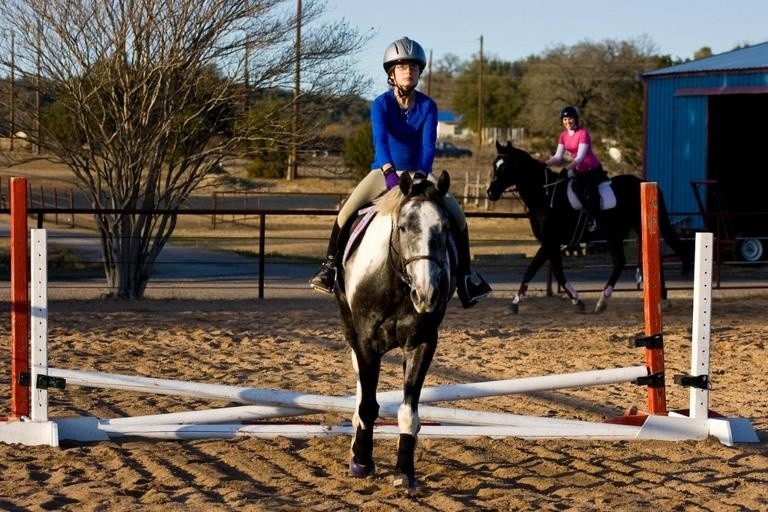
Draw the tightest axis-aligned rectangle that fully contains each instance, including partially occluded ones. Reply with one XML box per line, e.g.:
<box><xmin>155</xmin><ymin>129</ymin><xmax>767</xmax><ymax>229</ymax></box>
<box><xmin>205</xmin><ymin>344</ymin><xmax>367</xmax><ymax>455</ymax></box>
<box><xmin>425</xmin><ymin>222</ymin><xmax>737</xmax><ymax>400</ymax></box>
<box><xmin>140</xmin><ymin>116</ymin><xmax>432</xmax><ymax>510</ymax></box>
<box><xmin>6</xmin><ymin>26</ymin><xmax>19</xmax><ymax>150</ymax></box>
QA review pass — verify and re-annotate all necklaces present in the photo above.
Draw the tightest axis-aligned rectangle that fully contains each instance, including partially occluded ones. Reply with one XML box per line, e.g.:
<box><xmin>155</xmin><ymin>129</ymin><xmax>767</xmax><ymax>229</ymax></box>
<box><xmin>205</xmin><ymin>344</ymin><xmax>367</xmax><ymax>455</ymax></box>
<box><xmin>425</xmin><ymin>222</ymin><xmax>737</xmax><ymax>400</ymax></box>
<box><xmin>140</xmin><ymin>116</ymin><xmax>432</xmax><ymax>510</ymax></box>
<box><xmin>398</xmin><ymin>104</ymin><xmax>411</xmax><ymax>116</ymax></box>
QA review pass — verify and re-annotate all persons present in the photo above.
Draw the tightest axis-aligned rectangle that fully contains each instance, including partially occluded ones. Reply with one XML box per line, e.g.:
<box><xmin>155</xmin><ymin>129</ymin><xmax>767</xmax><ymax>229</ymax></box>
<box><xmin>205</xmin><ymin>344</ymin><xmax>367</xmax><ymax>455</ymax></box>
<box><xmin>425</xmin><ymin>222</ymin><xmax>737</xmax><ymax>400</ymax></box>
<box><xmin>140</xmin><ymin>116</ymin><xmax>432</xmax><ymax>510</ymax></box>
<box><xmin>545</xmin><ymin>107</ymin><xmax>610</xmax><ymax>233</ymax></box>
<box><xmin>308</xmin><ymin>38</ymin><xmax>490</xmax><ymax>309</ymax></box>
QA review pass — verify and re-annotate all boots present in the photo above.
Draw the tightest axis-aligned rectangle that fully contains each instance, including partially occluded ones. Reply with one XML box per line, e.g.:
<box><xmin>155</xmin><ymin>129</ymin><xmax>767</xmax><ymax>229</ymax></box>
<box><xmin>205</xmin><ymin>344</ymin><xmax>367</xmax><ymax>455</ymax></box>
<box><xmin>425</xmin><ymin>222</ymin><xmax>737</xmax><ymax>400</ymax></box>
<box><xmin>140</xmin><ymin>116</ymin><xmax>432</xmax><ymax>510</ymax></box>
<box><xmin>452</xmin><ymin>223</ymin><xmax>488</xmax><ymax>309</ymax></box>
<box><xmin>310</xmin><ymin>219</ymin><xmax>344</xmax><ymax>291</ymax></box>
<box><xmin>585</xmin><ymin>195</ymin><xmax>600</xmax><ymax>230</ymax></box>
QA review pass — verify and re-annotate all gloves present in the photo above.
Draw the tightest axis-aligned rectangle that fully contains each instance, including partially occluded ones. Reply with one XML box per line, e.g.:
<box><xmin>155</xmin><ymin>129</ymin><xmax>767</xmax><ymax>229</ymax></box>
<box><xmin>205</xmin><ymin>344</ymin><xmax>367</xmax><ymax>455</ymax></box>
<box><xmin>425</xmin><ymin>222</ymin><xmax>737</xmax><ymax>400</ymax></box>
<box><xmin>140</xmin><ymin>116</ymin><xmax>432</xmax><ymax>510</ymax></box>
<box><xmin>383</xmin><ymin>166</ymin><xmax>400</xmax><ymax>189</ymax></box>
<box><xmin>412</xmin><ymin>170</ymin><xmax>428</xmax><ymax>192</ymax></box>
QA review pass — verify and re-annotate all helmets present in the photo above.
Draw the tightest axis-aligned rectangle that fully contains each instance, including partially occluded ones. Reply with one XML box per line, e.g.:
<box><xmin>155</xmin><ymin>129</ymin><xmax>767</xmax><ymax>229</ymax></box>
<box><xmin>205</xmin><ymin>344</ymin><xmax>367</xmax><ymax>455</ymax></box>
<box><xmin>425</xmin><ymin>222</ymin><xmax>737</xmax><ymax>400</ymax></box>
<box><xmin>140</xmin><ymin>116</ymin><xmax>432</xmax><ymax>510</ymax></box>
<box><xmin>560</xmin><ymin>106</ymin><xmax>579</xmax><ymax>121</ymax></box>
<box><xmin>382</xmin><ymin>37</ymin><xmax>427</xmax><ymax>74</ymax></box>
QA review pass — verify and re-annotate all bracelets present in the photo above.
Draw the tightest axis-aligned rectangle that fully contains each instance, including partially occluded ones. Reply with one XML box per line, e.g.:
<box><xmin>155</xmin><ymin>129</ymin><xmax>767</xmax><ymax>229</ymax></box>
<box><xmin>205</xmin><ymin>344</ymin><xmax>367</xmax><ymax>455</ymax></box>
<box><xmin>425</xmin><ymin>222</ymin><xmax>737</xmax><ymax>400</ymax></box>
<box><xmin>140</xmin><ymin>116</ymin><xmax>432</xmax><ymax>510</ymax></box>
<box><xmin>383</xmin><ymin>167</ymin><xmax>396</xmax><ymax>176</ymax></box>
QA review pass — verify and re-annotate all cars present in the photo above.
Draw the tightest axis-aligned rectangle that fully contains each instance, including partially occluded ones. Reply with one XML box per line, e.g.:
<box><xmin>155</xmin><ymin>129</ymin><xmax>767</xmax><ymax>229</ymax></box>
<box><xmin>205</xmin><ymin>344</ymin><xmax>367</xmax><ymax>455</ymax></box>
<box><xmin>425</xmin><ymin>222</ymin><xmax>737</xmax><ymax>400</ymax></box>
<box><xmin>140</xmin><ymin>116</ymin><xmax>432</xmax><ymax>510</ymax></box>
<box><xmin>310</xmin><ymin>136</ymin><xmax>345</xmax><ymax>157</ymax></box>
<box><xmin>434</xmin><ymin>138</ymin><xmax>473</xmax><ymax>159</ymax></box>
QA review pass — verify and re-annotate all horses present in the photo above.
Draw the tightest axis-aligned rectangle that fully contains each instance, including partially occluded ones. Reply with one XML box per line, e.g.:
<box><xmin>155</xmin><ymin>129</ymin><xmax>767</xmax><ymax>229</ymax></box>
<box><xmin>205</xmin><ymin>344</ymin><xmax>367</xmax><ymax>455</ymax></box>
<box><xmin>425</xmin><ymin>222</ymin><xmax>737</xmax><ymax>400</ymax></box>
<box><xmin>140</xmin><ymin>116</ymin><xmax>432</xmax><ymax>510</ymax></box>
<box><xmin>486</xmin><ymin>139</ymin><xmax>694</xmax><ymax>315</ymax></box>
<box><xmin>332</xmin><ymin>168</ymin><xmax>459</xmax><ymax>490</ymax></box>
